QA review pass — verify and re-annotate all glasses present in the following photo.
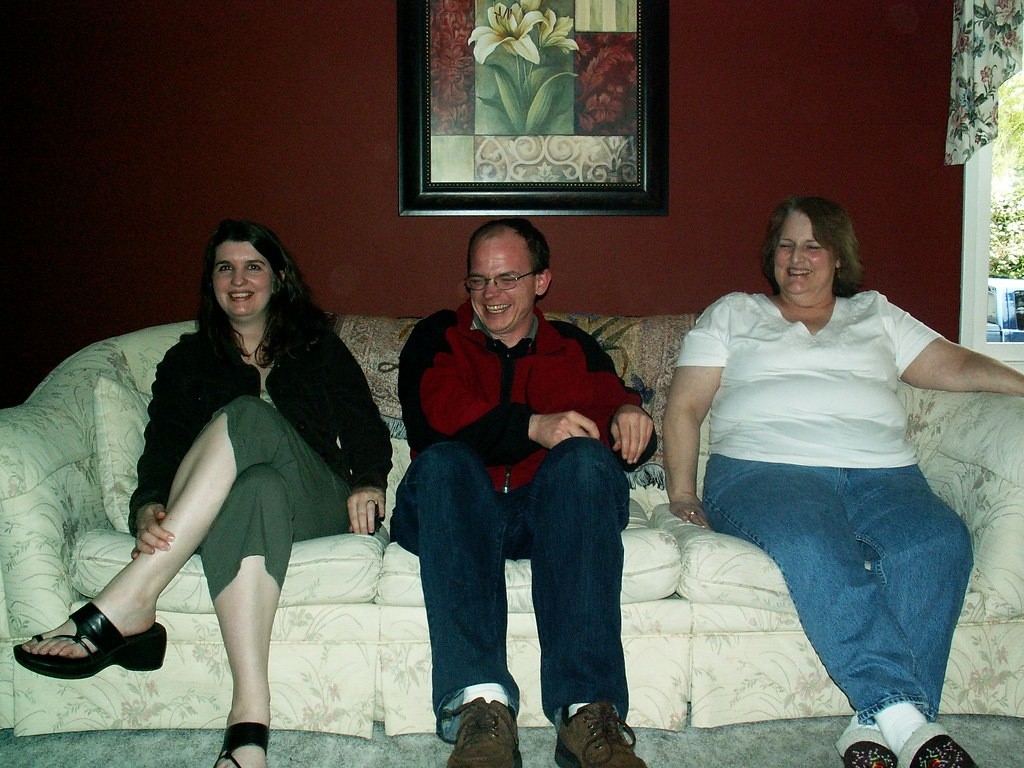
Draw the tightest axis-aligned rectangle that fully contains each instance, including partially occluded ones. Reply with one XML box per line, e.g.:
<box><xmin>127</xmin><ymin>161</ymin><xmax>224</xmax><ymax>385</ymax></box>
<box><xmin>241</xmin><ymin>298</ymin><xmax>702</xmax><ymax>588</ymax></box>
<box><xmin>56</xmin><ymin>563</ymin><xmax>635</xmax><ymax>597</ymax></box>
<box><xmin>463</xmin><ymin>271</ymin><xmax>536</xmax><ymax>290</ymax></box>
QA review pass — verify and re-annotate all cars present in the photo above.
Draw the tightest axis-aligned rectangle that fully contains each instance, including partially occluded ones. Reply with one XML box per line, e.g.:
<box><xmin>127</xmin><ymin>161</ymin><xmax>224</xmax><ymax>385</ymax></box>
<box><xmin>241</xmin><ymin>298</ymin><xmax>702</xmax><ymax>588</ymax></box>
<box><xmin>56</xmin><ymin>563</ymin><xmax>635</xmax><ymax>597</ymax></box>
<box><xmin>986</xmin><ymin>278</ymin><xmax>1024</xmax><ymax>342</ymax></box>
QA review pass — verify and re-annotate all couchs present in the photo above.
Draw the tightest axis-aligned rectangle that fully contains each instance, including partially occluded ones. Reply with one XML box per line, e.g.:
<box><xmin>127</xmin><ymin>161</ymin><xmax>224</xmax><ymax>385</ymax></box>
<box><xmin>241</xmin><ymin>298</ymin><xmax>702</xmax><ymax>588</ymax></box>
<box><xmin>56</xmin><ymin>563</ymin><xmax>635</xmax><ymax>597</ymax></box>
<box><xmin>0</xmin><ymin>313</ymin><xmax>1024</xmax><ymax>740</ymax></box>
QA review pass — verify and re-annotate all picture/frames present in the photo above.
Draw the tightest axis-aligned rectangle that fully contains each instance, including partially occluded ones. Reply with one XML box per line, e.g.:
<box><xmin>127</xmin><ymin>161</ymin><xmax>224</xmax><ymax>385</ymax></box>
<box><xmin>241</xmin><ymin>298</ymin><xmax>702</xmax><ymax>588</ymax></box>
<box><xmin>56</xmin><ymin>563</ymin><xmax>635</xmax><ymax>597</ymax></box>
<box><xmin>396</xmin><ymin>0</ymin><xmax>671</xmax><ymax>216</ymax></box>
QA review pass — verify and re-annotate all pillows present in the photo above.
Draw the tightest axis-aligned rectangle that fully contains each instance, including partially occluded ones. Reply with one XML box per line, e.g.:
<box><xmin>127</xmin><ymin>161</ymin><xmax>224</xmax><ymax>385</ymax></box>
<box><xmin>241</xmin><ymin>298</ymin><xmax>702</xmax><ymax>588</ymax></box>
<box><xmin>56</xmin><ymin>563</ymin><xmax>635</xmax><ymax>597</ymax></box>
<box><xmin>93</xmin><ymin>377</ymin><xmax>153</xmax><ymax>536</ymax></box>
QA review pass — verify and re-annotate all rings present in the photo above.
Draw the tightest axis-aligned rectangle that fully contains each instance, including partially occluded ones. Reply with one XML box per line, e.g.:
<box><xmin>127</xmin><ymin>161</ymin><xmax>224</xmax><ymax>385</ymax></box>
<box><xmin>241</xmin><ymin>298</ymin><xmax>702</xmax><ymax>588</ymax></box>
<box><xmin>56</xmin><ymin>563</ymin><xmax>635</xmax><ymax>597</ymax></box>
<box><xmin>365</xmin><ymin>500</ymin><xmax>376</xmax><ymax>506</ymax></box>
<box><xmin>688</xmin><ymin>512</ymin><xmax>697</xmax><ymax>517</ymax></box>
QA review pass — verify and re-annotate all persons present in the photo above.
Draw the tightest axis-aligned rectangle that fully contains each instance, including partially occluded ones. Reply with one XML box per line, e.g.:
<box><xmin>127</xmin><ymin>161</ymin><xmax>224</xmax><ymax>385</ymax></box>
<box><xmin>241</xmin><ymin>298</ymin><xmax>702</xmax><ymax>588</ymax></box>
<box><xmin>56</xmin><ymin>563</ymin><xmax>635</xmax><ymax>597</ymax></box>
<box><xmin>14</xmin><ymin>218</ymin><xmax>395</xmax><ymax>768</ymax></box>
<box><xmin>389</xmin><ymin>218</ymin><xmax>657</xmax><ymax>768</ymax></box>
<box><xmin>663</xmin><ymin>196</ymin><xmax>1024</xmax><ymax>768</ymax></box>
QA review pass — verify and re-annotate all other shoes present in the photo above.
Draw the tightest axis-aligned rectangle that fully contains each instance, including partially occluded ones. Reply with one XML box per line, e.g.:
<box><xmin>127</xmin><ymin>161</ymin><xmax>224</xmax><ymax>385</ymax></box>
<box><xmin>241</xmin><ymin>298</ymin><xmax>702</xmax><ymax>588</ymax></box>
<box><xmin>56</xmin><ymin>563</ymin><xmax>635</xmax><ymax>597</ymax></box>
<box><xmin>896</xmin><ymin>722</ymin><xmax>979</xmax><ymax>768</ymax></box>
<box><xmin>834</xmin><ymin>727</ymin><xmax>899</xmax><ymax>768</ymax></box>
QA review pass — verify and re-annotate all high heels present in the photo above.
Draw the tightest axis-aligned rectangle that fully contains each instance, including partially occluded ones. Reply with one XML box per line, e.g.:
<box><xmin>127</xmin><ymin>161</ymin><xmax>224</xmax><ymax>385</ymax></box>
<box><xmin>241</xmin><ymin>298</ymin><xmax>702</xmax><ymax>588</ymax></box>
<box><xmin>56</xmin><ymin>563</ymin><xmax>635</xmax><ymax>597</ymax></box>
<box><xmin>213</xmin><ymin>722</ymin><xmax>269</xmax><ymax>768</ymax></box>
<box><xmin>13</xmin><ymin>601</ymin><xmax>167</xmax><ymax>679</ymax></box>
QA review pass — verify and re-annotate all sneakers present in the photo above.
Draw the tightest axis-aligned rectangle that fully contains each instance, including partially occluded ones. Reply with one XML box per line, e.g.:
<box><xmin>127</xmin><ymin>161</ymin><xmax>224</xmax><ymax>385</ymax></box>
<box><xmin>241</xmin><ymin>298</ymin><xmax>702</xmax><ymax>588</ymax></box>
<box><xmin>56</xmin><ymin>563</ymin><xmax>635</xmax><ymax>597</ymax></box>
<box><xmin>555</xmin><ymin>702</ymin><xmax>647</xmax><ymax>768</ymax></box>
<box><xmin>447</xmin><ymin>697</ymin><xmax>521</xmax><ymax>768</ymax></box>
